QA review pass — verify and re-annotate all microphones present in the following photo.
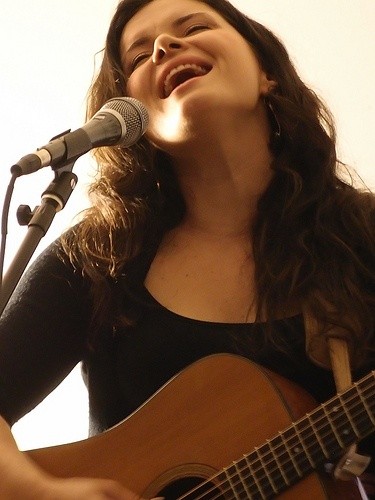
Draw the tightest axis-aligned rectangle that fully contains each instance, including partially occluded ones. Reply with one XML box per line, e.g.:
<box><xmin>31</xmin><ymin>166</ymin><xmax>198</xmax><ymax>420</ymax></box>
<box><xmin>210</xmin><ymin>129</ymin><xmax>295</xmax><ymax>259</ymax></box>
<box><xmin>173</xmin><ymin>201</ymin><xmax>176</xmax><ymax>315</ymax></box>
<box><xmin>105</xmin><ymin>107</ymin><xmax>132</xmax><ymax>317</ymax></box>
<box><xmin>11</xmin><ymin>97</ymin><xmax>149</xmax><ymax>177</ymax></box>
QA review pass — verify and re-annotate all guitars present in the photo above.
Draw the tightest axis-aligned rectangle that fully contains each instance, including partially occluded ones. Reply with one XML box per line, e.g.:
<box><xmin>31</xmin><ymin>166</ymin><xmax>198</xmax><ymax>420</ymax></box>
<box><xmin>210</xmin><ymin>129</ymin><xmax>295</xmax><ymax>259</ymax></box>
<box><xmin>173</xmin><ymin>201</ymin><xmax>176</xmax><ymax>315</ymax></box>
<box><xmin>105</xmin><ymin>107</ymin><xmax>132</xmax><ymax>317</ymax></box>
<box><xmin>20</xmin><ymin>351</ymin><xmax>375</xmax><ymax>500</ymax></box>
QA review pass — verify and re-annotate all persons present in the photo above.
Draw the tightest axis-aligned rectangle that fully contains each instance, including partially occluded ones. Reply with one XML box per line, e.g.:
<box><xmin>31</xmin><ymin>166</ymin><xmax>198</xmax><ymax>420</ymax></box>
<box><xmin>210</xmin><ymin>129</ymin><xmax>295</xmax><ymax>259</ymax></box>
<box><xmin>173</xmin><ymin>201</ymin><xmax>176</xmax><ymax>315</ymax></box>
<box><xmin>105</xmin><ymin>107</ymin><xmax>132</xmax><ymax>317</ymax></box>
<box><xmin>0</xmin><ymin>0</ymin><xmax>375</xmax><ymax>500</ymax></box>
